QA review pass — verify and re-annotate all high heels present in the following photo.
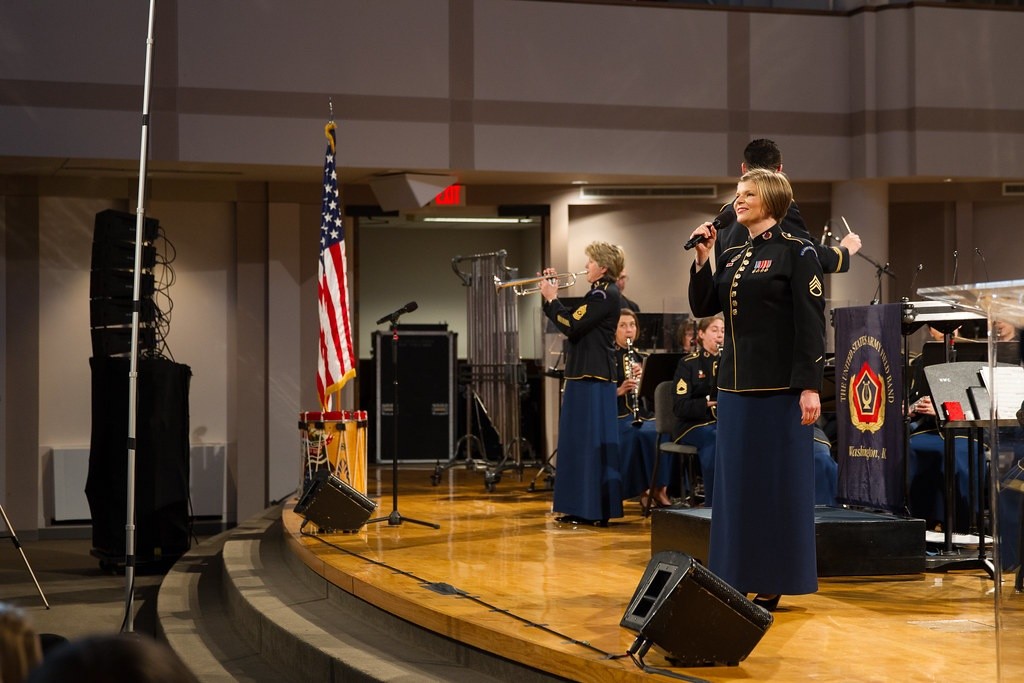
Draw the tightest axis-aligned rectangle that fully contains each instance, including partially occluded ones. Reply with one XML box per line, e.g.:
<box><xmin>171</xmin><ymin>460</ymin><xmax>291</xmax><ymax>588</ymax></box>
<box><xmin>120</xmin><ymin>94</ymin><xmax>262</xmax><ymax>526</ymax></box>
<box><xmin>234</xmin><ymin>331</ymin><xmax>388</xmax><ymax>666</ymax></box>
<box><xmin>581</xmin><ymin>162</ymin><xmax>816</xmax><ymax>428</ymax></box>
<box><xmin>555</xmin><ymin>514</ymin><xmax>608</xmax><ymax>528</ymax></box>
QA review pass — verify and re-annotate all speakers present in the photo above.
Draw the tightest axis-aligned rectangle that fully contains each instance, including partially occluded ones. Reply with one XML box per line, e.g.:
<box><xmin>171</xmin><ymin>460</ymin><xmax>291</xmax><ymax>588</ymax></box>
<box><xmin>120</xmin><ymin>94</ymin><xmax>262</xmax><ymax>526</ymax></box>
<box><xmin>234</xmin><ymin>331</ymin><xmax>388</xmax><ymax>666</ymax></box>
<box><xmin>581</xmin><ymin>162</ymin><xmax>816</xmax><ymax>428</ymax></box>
<box><xmin>294</xmin><ymin>468</ymin><xmax>377</xmax><ymax>532</ymax></box>
<box><xmin>620</xmin><ymin>549</ymin><xmax>774</xmax><ymax>669</ymax></box>
<box><xmin>87</xmin><ymin>208</ymin><xmax>189</xmax><ymax>577</ymax></box>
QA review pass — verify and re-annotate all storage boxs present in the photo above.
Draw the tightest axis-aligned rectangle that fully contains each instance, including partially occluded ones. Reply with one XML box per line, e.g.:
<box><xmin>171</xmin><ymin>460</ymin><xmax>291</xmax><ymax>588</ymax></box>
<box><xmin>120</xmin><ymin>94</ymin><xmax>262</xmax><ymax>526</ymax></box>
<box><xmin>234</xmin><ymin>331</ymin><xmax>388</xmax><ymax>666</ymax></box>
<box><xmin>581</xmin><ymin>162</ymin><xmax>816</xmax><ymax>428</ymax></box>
<box><xmin>375</xmin><ymin>330</ymin><xmax>457</xmax><ymax>464</ymax></box>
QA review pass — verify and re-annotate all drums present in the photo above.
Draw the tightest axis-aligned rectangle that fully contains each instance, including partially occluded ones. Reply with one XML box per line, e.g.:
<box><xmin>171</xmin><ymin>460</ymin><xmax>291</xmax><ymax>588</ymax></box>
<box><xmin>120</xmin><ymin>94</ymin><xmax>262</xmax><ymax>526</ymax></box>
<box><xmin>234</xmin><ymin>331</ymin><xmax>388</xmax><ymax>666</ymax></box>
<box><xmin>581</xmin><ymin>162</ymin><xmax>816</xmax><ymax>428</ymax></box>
<box><xmin>517</xmin><ymin>362</ymin><xmax>539</xmax><ymax>400</ymax></box>
<box><xmin>301</xmin><ymin>410</ymin><xmax>369</xmax><ymax>532</ymax></box>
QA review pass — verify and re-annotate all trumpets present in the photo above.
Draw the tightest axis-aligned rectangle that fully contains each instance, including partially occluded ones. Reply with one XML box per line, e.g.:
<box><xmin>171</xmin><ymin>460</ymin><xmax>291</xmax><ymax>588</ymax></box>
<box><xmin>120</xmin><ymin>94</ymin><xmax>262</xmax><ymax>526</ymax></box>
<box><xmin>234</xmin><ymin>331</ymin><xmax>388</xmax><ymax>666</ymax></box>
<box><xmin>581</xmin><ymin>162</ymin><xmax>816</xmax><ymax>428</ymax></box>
<box><xmin>493</xmin><ymin>270</ymin><xmax>590</xmax><ymax>297</ymax></box>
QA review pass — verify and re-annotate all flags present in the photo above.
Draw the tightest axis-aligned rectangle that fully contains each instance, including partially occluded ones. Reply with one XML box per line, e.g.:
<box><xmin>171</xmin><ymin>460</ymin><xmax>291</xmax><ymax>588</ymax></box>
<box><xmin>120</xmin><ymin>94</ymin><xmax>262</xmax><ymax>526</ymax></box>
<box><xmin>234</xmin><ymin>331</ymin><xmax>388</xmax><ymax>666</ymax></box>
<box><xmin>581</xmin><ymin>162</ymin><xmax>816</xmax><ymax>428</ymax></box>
<box><xmin>316</xmin><ymin>121</ymin><xmax>357</xmax><ymax>413</ymax></box>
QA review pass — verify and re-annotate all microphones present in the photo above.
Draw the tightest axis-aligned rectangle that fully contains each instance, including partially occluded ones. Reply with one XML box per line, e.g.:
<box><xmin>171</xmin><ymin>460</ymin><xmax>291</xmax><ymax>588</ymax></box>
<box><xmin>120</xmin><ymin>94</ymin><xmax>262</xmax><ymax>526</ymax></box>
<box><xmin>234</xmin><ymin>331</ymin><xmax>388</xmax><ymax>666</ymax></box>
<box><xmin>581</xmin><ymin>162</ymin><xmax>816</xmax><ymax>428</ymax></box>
<box><xmin>820</xmin><ymin>221</ymin><xmax>829</xmax><ymax>245</ymax></box>
<box><xmin>683</xmin><ymin>210</ymin><xmax>734</xmax><ymax>250</ymax></box>
<box><xmin>376</xmin><ymin>302</ymin><xmax>418</xmax><ymax>324</ymax></box>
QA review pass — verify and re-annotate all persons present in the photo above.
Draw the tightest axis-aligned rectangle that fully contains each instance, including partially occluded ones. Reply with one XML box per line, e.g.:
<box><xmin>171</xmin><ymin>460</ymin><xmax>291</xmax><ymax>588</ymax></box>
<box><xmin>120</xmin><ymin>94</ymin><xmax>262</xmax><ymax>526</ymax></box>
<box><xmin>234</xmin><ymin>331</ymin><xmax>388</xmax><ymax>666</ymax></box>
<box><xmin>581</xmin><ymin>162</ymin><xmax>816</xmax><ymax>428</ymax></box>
<box><xmin>663</xmin><ymin>314</ymin><xmax>696</xmax><ymax>352</ymax></box>
<box><xmin>612</xmin><ymin>308</ymin><xmax>691</xmax><ymax>512</ymax></box>
<box><xmin>689</xmin><ymin>169</ymin><xmax>827</xmax><ymax>611</ymax></box>
<box><xmin>902</xmin><ymin>320</ymin><xmax>991</xmax><ymax>556</ymax></box>
<box><xmin>670</xmin><ymin>316</ymin><xmax>725</xmax><ymax>507</ymax></box>
<box><xmin>537</xmin><ymin>242</ymin><xmax>624</xmax><ymax>527</ymax></box>
<box><xmin>714</xmin><ymin>139</ymin><xmax>862</xmax><ymax>274</ymax></box>
<box><xmin>994</xmin><ymin>320</ymin><xmax>1024</xmax><ymax>571</ymax></box>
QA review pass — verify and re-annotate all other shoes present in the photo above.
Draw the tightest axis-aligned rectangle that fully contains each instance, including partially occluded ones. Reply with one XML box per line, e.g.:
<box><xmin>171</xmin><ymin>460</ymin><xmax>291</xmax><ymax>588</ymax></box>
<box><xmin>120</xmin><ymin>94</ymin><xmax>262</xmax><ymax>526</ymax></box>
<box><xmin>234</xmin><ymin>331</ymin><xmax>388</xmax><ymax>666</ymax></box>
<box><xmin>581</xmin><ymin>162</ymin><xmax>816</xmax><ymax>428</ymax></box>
<box><xmin>751</xmin><ymin>594</ymin><xmax>781</xmax><ymax>610</ymax></box>
<box><xmin>639</xmin><ymin>497</ymin><xmax>658</xmax><ymax>514</ymax></box>
<box><xmin>656</xmin><ymin>496</ymin><xmax>673</xmax><ymax>509</ymax></box>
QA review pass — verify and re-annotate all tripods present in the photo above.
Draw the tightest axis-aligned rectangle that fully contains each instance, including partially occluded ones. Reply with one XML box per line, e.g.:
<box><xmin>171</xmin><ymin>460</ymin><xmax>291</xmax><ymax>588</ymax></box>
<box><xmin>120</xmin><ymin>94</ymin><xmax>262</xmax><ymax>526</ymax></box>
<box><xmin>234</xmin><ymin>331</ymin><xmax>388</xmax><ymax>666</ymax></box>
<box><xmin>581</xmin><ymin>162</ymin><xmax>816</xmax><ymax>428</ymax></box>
<box><xmin>365</xmin><ymin>318</ymin><xmax>440</xmax><ymax>529</ymax></box>
<box><xmin>923</xmin><ymin>360</ymin><xmax>1024</xmax><ymax>579</ymax></box>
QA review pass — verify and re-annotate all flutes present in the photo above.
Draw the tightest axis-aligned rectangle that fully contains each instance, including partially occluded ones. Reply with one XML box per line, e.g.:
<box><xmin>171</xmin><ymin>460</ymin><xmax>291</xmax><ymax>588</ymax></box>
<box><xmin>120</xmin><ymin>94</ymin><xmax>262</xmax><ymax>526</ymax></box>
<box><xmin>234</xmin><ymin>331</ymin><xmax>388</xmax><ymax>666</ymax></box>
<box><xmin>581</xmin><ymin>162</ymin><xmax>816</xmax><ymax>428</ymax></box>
<box><xmin>623</xmin><ymin>336</ymin><xmax>644</xmax><ymax>428</ymax></box>
<box><xmin>716</xmin><ymin>341</ymin><xmax>724</xmax><ymax>358</ymax></box>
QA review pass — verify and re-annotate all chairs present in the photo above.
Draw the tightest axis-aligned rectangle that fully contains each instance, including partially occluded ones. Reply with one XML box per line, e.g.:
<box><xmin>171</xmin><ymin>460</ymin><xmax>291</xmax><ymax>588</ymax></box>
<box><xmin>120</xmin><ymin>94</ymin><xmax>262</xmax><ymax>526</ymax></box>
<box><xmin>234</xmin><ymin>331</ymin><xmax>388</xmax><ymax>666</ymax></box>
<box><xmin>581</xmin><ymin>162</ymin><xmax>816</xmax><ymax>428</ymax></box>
<box><xmin>645</xmin><ymin>381</ymin><xmax>698</xmax><ymax>516</ymax></box>
